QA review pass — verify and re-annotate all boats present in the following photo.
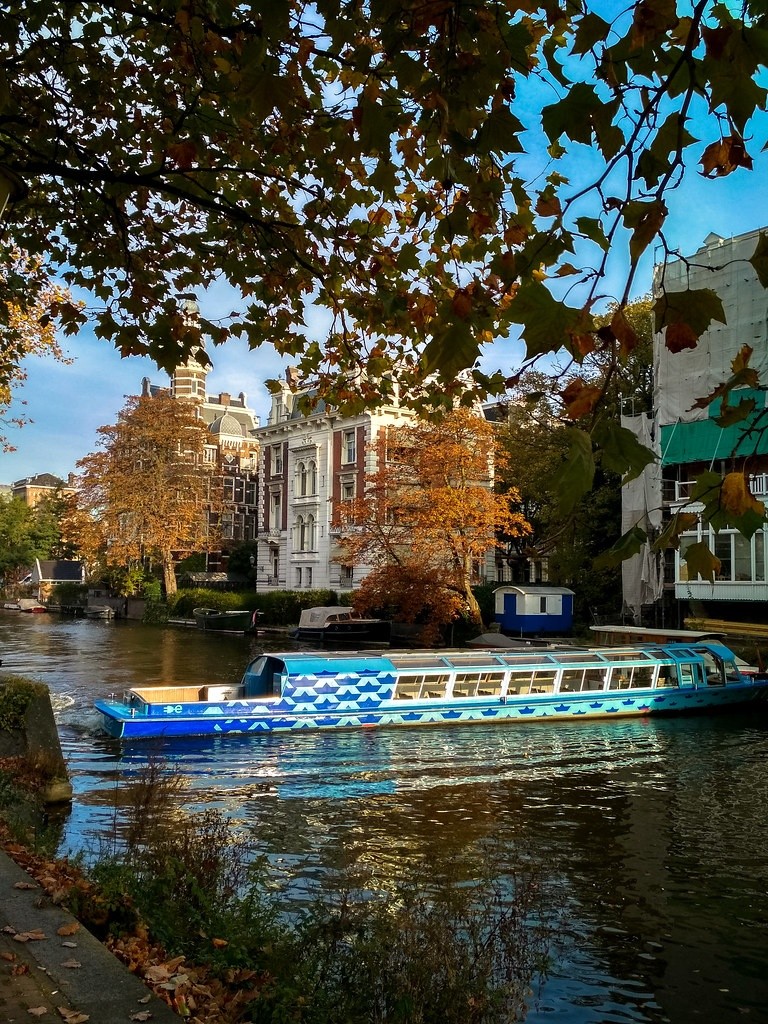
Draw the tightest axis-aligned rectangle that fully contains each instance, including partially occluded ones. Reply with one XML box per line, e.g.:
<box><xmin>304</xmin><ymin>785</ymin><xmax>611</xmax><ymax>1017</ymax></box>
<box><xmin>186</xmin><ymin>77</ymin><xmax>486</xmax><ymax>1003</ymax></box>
<box><xmin>551</xmin><ymin>615</ymin><xmax>759</xmax><ymax>677</ymax></box>
<box><xmin>286</xmin><ymin>606</ymin><xmax>392</xmax><ymax>649</ymax></box>
<box><xmin>193</xmin><ymin>607</ymin><xmax>260</xmax><ymax>633</ymax></box>
<box><xmin>83</xmin><ymin>605</ymin><xmax>116</xmax><ymax>619</ymax></box>
<box><xmin>16</xmin><ymin>599</ymin><xmax>47</xmax><ymax>613</ymax></box>
<box><xmin>94</xmin><ymin>639</ymin><xmax>768</xmax><ymax>739</ymax></box>
